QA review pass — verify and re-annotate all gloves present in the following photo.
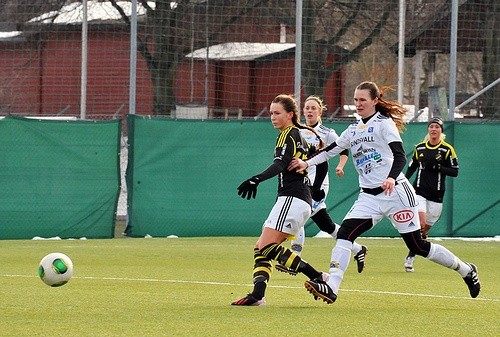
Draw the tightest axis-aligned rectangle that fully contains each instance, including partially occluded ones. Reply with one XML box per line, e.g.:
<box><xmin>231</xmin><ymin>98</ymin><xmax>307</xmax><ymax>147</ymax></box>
<box><xmin>237</xmin><ymin>176</ymin><xmax>261</xmax><ymax>200</ymax></box>
<box><xmin>311</xmin><ymin>189</ymin><xmax>325</xmax><ymax>202</ymax></box>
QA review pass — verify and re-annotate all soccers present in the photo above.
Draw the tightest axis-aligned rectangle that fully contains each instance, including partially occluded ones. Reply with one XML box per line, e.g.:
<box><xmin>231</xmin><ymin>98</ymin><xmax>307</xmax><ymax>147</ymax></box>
<box><xmin>39</xmin><ymin>253</ymin><xmax>73</xmax><ymax>287</ymax></box>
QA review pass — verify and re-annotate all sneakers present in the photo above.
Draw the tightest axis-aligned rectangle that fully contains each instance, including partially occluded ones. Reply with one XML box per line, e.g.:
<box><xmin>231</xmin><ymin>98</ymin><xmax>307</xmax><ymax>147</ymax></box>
<box><xmin>230</xmin><ymin>294</ymin><xmax>268</xmax><ymax>306</ymax></box>
<box><xmin>404</xmin><ymin>256</ymin><xmax>414</xmax><ymax>272</ymax></box>
<box><xmin>311</xmin><ymin>270</ymin><xmax>328</xmax><ymax>301</ymax></box>
<box><xmin>354</xmin><ymin>246</ymin><xmax>368</xmax><ymax>273</ymax></box>
<box><xmin>304</xmin><ymin>279</ymin><xmax>338</xmax><ymax>304</ymax></box>
<box><xmin>462</xmin><ymin>262</ymin><xmax>480</xmax><ymax>298</ymax></box>
<box><xmin>275</xmin><ymin>263</ymin><xmax>298</xmax><ymax>276</ymax></box>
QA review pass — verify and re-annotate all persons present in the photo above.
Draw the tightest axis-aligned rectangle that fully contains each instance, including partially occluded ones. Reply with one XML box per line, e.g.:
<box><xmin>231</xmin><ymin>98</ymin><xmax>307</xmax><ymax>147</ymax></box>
<box><xmin>276</xmin><ymin>96</ymin><xmax>368</xmax><ymax>276</ymax></box>
<box><xmin>231</xmin><ymin>94</ymin><xmax>329</xmax><ymax>306</ymax></box>
<box><xmin>287</xmin><ymin>81</ymin><xmax>480</xmax><ymax>304</ymax></box>
<box><xmin>406</xmin><ymin>118</ymin><xmax>458</xmax><ymax>273</ymax></box>
